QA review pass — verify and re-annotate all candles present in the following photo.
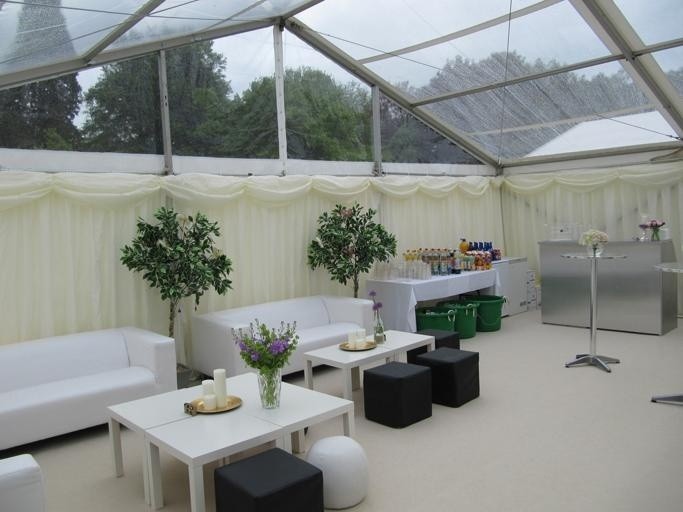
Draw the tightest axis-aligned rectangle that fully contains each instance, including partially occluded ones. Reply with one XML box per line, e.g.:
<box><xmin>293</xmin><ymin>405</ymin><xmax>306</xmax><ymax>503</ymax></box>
<box><xmin>348</xmin><ymin>327</ymin><xmax>356</xmax><ymax>349</ymax></box>
<box><xmin>201</xmin><ymin>379</ymin><xmax>213</xmax><ymax>395</ymax></box>
<box><xmin>201</xmin><ymin>393</ymin><xmax>217</xmax><ymax>411</ymax></box>
<box><xmin>355</xmin><ymin>337</ymin><xmax>365</xmax><ymax>349</ymax></box>
<box><xmin>213</xmin><ymin>368</ymin><xmax>228</xmax><ymax>409</ymax></box>
<box><xmin>357</xmin><ymin>328</ymin><xmax>365</xmax><ymax>338</ymax></box>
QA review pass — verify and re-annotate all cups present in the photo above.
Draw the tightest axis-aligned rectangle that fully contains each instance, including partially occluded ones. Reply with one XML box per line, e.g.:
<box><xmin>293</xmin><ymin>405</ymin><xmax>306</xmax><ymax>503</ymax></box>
<box><xmin>347</xmin><ymin>328</ymin><xmax>367</xmax><ymax>346</ymax></box>
<box><xmin>201</xmin><ymin>368</ymin><xmax>227</xmax><ymax>409</ymax></box>
<box><xmin>368</xmin><ymin>258</ymin><xmax>432</xmax><ymax>282</ymax></box>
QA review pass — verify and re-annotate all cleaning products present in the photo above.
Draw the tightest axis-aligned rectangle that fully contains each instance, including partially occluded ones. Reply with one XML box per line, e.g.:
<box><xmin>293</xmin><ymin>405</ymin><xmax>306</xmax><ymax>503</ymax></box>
<box><xmin>469</xmin><ymin>240</ymin><xmax>502</xmax><ymax>259</ymax></box>
<box><xmin>370</xmin><ymin>248</ymin><xmax>492</xmax><ymax>281</ymax></box>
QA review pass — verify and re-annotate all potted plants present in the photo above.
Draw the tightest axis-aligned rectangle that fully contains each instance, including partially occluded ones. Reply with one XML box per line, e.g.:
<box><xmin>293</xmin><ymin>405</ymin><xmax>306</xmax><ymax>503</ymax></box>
<box><xmin>116</xmin><ymin>202</ymin><xmax>234</xmax><ymax>391</ymax></box>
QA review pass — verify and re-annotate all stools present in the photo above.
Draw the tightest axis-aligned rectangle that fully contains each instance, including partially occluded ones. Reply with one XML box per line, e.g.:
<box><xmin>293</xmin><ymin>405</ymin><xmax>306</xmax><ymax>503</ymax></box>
<box><xmin>416</xmin><ymin>345</ymin><xmax>479</xmax><ymax>409</ymax></box>
<box><xmin>0</xmin><ymin>452</ymin><xmax>47</xmax><ymax>512</ymax></box>
<box><xmin>212</xmin><ymin>446</ymin><xmax>323</xmax><ymax>512</ymax></box>
<box><xmin>361</xmin><ymin>360</ymin><xmax>432</xmax><ymax>429</ymax></box>
<box><xmin>405</xmin><ymin>327</ymin><xmax>461</xmax><ymax>367</ymax></box>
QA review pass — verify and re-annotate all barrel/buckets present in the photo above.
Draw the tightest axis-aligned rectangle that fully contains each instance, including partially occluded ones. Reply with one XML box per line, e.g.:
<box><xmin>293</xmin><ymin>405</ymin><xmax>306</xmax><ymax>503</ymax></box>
<box><xmin>437</xmin><ymin>298</ymin><xmax>480</xmax><ymax>338</ymax></box>
<box><xmin>464</xmin><ymin>294</ymin><xmax>506</xmax><ymax>332</ymax></box>
<box><xmin>416</xmin><ymin>304</ymin><xmax>457</xmax><ymax>331</ymax></box>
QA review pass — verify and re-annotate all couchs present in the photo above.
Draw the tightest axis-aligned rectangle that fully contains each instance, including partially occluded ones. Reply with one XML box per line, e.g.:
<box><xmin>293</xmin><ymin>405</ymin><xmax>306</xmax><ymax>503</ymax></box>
<box><xmin>0</xmin><ymin>323</ymin><xmax>178</xmax><ymax>453</ymax></box>
<box><xmin>188</xmin><ymin>293</ymin><xmax>376</xmax><ymax>382</ymax></box>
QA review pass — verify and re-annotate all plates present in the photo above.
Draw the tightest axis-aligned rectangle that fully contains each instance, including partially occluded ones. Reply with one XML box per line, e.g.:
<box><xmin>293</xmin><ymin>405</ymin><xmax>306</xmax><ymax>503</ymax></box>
<box><xmin>338</xmin><ymin>342</ymin><xmax>376</xmax><ymax>351</ymax></box>
<box><xmin>187</xmin><ymin>394</ymin><xmax>244</xmax><ymax>414</ymax></box>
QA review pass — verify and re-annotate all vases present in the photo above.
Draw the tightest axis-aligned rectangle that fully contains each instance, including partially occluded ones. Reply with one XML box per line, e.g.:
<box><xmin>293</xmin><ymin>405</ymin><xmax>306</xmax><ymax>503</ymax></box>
<box><xmin>585</xmin><ymin>240</ymin><xmax>605</xmax><ymax>257</ymax></box>
<box><xmin>650</xmin><ymin>227</ymin><xmax>661</xmax><ymax>241</ymax></box>
<box><xmin>256</xmin><ymin>366</ymin><xmax>281</xmax><ymax>410</ymax></box>
<box><xmin>371</xmin><ymin>310</ymin><xmax>384</xmax><ymax>345</ymax></box>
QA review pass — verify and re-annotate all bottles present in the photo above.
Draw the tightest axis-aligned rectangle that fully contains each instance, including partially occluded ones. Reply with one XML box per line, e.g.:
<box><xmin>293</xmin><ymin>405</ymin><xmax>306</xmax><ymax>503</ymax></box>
<box><xmin>403</xmin><ymin>237</ymin><xmax>502</xmax><ymax>280</ymax></box>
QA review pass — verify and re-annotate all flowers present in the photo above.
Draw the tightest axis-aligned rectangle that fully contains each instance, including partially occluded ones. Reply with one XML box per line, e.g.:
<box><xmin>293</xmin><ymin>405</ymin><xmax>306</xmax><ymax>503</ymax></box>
<box><xmin>579</xmin><ymin>228</ymin><xmax>609</xmax><ymax>248</ymax></box>
<box><xmin>229</xmin><ymin>317</ymin><xmax>300</xmax><ymax>407</ymax></box>
<box><xmin>368</xmin><ymin>289</ymin><xmax>384</xmax><ymax>342</ymax></box>
<box><xmin>646</xmin><ymin>218</ymin><xmax>667</xmax><ymax>240</ymax></box>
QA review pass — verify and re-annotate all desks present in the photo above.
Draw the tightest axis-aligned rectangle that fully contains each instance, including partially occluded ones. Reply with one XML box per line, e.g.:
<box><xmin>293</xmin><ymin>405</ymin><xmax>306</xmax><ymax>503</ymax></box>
<box><xmin>228</xmin><ymin>381</ymin><xmax>356</xmax><ymax>455</ymax></box>
<box><xmin>645</xmin><ymin>260</ymin><xmax>683</xmax><ymax>404</ymax></box>
<box><xmin>364</xmin><ymin>266</ymin><xmax>497</xmax><ymax>363</ymax></box>
<box><xmin>558</xmin><ymin>251</ymin><xmax>626</xmax><ymax>372</ymax></box>
<box><xmin>301</xmin><ymin>336</ymin><xmax>395</xmax><ymax>403</ymax></box>
<box><xmin>364</xmin><ymin>329</ymin><xmax>437</xmax><ymax>366</ymax></box>
<box><xmin>104</xmin><ymin>390</ymin><xmax>208</xmax><ymax>507</ymax></box>
<box><xmin>143</xmin><ymin>403</ymin><xmax>285</xmax><ymax>512</ymax></box>
<box><xmin>186</xmin><ymin>370</ymin><xmax>285</xmax><ymax>406</ymax></box>
